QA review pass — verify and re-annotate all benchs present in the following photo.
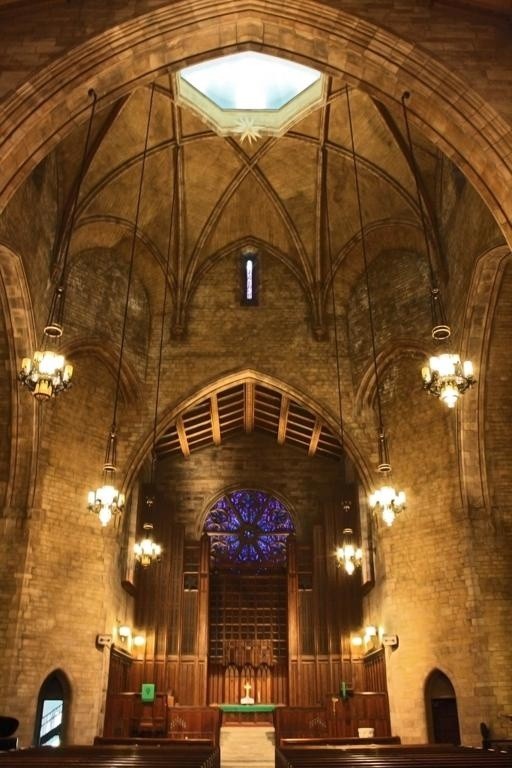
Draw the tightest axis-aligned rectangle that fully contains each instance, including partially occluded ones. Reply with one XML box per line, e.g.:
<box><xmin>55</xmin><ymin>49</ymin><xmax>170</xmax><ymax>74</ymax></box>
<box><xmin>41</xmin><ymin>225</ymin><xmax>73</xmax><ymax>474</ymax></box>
<box><xmin>275</xmin><ymin>745</ymin><xmax>512</xmax><ymax>768</ymax></box>
<box><xmin>0</xmin><ymin>745</ymin><xmax>220</xmax><ymax>767</ymax></box>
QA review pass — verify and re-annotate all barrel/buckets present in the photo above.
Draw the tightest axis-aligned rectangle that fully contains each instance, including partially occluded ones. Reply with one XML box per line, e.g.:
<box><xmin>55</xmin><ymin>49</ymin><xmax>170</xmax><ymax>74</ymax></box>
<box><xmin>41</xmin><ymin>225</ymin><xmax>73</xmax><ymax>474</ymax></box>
<box><xmin>358</xmin><ymin>727</ymin><xmax>374</xmax><ymax>739</ymax></box>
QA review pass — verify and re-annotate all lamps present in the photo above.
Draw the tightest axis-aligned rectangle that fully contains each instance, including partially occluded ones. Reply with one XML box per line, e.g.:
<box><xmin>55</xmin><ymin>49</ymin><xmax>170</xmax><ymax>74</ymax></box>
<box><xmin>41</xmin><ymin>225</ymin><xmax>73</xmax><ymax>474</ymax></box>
<box><xmin>323</xmin><ymin>197</ymin><xmax>365</xmax><ymax>578</ymax></box>
<box><xmin>346</xmin><ymin>86</ymin><xmax>409</xmax><ymax>532</ymax></box>
<box><xmin>132</xmin><ymin>219</ymin><xmax>174</xmax><ymax>568</ymax></box>
<box><xmin>399</xmin><ymin>98</ymin><xmax>475</xmax><ymax>412</ymax></box>
<box><xmin>20</xmin><ymin>105</ymin><xmax>95</xmax><ymax>418</ymax></box>
<box><xmin>87</xmin><ymin>126</ymin><xmax>156</xmax><ymax>529</ymax></box>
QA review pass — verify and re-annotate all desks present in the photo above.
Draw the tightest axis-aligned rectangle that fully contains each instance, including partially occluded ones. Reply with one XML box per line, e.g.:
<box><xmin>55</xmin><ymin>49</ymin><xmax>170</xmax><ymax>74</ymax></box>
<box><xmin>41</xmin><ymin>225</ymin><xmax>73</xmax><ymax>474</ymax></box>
<box><xmin>117</xmin><ymin>693</ymin><xmax>221</xmax><ymax>740</ymax></box>
<box><xmin>276</xmin><ymin>691</ymin><xmax>392</xmax><ymax>737</ymax></box>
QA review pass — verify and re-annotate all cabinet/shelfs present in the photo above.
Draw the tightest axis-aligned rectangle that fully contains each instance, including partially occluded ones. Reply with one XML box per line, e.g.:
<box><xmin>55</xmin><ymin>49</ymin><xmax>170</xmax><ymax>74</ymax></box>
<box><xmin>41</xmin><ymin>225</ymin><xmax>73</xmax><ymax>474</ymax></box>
<box><xmin>210</xmin><ymin>702</ymin><xmax>282</xmax><ymax>723</ymax></box>
